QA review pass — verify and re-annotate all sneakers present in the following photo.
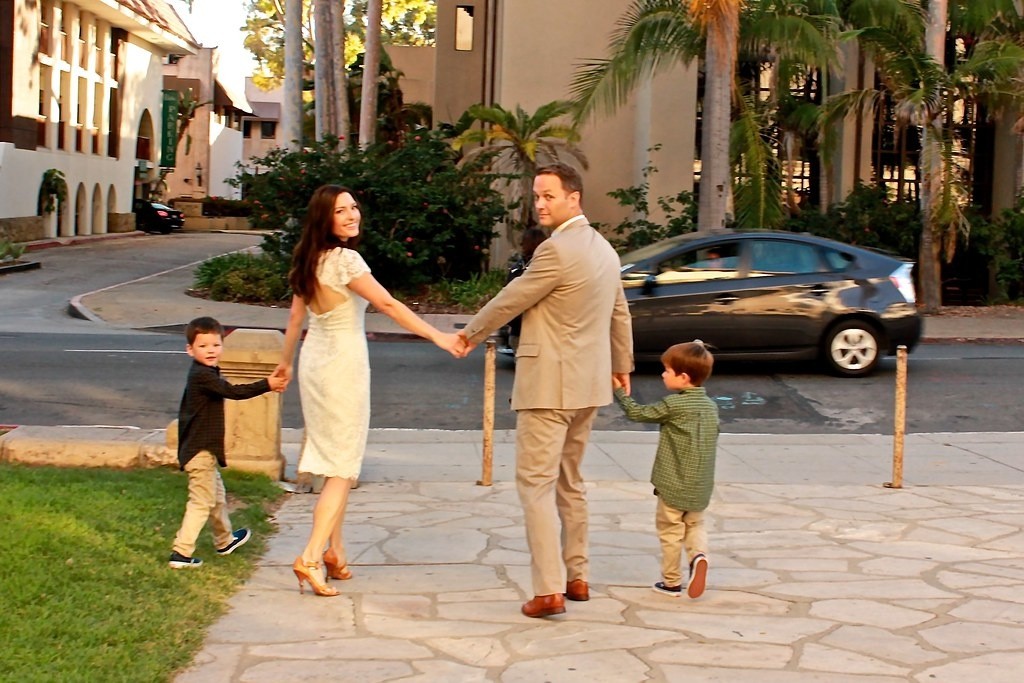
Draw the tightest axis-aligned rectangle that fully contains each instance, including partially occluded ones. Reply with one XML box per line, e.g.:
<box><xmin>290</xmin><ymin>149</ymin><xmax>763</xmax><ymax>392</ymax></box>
<box><xmin>653</xmin><ymin>582</ymin><xmax>682</xmax><ymax>598</ymax></box>
<box><xmin>686</xmin><ymin>554</ymin><xmax>707</xmax><ymax>599</ymax></box>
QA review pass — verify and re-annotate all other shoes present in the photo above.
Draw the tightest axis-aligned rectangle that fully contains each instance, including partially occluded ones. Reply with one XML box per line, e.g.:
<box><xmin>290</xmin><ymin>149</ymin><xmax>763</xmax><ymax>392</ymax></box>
<box><xmin>217</xmin><ymin>527</ymin><xmax>251</xmax><ymax>555</ymax></box>
<box><xmin>168</xmin><ymin>551</ymin><xmax>203</xmax><ymax>569</ymax></box>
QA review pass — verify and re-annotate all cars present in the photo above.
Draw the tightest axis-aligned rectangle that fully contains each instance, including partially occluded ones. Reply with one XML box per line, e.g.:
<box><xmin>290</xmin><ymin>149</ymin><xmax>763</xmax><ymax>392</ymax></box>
<box><xmin>487</xmin><ymin>229</ymin><xmax>924</xmax><ymax>376</ymax></box>
<box><xmin>133</xmin><ymin>198</ymin><xmax>186</xmax><ymax>235</ymax></box>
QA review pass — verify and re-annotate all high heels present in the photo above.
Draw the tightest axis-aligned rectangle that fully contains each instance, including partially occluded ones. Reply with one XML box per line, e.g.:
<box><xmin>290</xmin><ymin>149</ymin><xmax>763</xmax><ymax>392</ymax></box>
<box><xmin>292</xmin><ymin>556</ymin><xmax>340</xmax><ymax>597</ymax></box>
<box><xmin>323</xmin><ymin>546</ymin><xmax>353</xmax><ymax>583</ymax></box>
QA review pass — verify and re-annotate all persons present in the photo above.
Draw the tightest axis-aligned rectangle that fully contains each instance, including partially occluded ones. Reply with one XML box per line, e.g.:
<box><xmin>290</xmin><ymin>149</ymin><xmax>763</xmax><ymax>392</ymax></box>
<box><xmin>273</xmin><ymin>183</ymin><xmax>465</xmax><ymax>597</ymax></box>
<box><xmin>613</xmin><ymin>342</ymin><xmax>719</xmax><ymax>598</ymax></box>
<box><xmin>169</xmin><ymin>316</ymin><xmax>286</xmax><ymax>568</ymax></box>
<box><xmin>455</xmin><ymin>162</ymin><xmax>633</xmax><ymax>617</ymax></box>
<box><xmin>661</xmin><ymin>247</ymin><xmax>729</xmax><ymax>274</ymax></box>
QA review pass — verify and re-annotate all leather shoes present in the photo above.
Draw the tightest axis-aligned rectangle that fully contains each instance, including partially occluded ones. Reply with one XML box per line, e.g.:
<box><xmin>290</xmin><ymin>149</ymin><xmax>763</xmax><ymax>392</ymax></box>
<box><xmin>566</xmin><ymin>580</ymin><xmax>590</xmax><ymax>601</ymax></box>
<box><xmin>522</xmin><ymin>593</ymin><xmax>567</xmax><ymax>618</ymax></box>
